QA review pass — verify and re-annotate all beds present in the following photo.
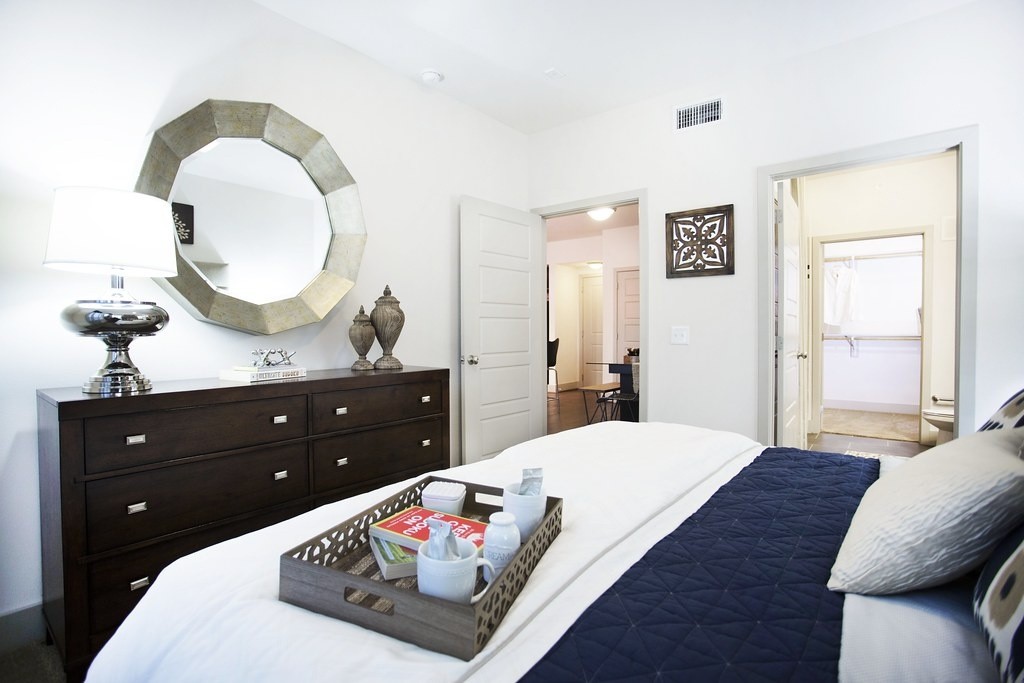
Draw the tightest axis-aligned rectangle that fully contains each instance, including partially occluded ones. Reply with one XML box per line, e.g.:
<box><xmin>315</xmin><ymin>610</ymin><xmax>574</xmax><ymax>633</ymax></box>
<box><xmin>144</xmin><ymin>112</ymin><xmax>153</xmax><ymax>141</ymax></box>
<box><xmin>82</xmin><ymin>422</ymin><xmax>1023</xmax><ymax>683</ymax></box>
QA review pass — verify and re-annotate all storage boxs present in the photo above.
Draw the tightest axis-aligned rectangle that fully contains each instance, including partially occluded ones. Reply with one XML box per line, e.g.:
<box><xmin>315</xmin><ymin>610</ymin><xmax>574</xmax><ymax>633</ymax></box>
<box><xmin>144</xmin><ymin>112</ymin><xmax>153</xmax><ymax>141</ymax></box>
<box><xmin>623</xmin><ymin>355</ymin><xmax>640</xmax><ymax>364</ymax></box>
<box><xmin>278</xmin><ymin>475</ymin><xmax>564</xmax><ymax>662</ymax></box>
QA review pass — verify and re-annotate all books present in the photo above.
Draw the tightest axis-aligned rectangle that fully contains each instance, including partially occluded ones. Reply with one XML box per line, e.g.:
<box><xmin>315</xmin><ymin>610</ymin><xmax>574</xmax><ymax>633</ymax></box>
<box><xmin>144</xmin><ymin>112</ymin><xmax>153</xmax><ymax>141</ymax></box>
<box><xmin>220</xmin><ymin>367</ymin><xmax>307</xmax><ymax>383</ymax></box>
<box><xmin>370</xmin><ymin>536</ymin><xmax>418</xmax><ymax>580</ymax></box>
<box><xmin>370</xmin><ymin>505</ymin><xmax>489</xmax><ymax>558</ymax></box>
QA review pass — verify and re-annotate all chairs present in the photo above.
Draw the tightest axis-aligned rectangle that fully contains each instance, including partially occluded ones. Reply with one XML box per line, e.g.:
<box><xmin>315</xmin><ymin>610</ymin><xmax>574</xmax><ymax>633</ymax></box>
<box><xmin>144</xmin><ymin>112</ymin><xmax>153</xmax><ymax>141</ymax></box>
<box><xmin>595</xmin><ymin>388</ymin><xmax>640</xmax><ymax>423</ymax></box>
<box><xmin>548</xmin><ymin>338</ymin><xmax>560</xmax><ymax>401</ymax></box>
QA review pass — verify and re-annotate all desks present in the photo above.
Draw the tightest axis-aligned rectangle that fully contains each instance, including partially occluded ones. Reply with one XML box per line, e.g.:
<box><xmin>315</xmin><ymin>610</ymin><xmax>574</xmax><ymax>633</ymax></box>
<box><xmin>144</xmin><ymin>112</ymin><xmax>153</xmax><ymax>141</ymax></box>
<box><xmin>577</xmin><ymin>382</ymin><xmax>621</xmax><ymax>425</ymax></box>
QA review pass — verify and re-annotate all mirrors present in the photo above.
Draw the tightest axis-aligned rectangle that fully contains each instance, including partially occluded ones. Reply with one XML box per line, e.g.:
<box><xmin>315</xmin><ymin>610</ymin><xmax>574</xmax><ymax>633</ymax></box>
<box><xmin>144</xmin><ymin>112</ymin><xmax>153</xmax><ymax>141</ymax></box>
<box><xmin>130</xmin><ymin>98</ymin><xmax>368</xmax><ymax>337</ymax></box>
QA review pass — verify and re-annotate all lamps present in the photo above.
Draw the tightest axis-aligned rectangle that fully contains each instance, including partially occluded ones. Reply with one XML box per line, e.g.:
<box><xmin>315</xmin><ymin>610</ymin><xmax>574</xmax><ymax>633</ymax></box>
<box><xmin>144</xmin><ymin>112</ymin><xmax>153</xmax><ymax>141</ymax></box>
<box><xmin>587</xmin><ymin>207</ymin><xmax>616</xmax><ymax>223</ymax></box>
<box><xmin>40</xmin><ymin>187</ymin><xmax>179</xmax><ymax>395</ymax></box>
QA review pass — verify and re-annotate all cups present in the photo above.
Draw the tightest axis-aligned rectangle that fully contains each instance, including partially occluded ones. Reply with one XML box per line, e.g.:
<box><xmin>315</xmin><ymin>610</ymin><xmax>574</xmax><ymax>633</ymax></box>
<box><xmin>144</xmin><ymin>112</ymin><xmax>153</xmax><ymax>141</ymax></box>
<box><xmin>500</xmin><ymin>482</ymin><xmax>546</xmax><ymax>545</ymax></box>
<box><xmin>415</xmin><ymin>535</ymin><xmax>495</xmax><ymax>606</ymax></box>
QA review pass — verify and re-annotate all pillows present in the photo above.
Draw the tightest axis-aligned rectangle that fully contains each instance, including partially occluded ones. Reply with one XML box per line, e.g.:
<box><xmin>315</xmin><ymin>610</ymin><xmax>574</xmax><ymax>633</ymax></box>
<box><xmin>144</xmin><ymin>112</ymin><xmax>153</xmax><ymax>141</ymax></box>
<box><xmin>970</xmin><ymin>524</ymin><xmax>1024</xmax><ymax>683</ymax></box>
<box><xmin>827</xmin><ymin>425</ymin><xmax>1024</xmax><ymax>596</ymax></box>
<box><xmin>978</xmin><ymin>387</ymin><xmax>1024</xmax><ymax>434</ymax></box>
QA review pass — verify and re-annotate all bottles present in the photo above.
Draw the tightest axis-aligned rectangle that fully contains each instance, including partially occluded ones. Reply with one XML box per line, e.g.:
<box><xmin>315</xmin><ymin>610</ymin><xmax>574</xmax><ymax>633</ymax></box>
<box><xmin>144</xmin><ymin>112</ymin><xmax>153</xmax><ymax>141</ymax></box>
<box><xmin>482</xmin><ymin>510</ymin><xmax>522</xmax><ymax>583</ymax></box>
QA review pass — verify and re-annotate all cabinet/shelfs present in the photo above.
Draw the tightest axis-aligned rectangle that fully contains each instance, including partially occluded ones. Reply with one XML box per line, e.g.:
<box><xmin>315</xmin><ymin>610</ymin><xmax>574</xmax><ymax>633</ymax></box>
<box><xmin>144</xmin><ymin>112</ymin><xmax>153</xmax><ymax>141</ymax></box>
<box><xmin>36</xmin><ymin>365</ymin><xmax>452</xmax><ymax>681</ymax></box>
